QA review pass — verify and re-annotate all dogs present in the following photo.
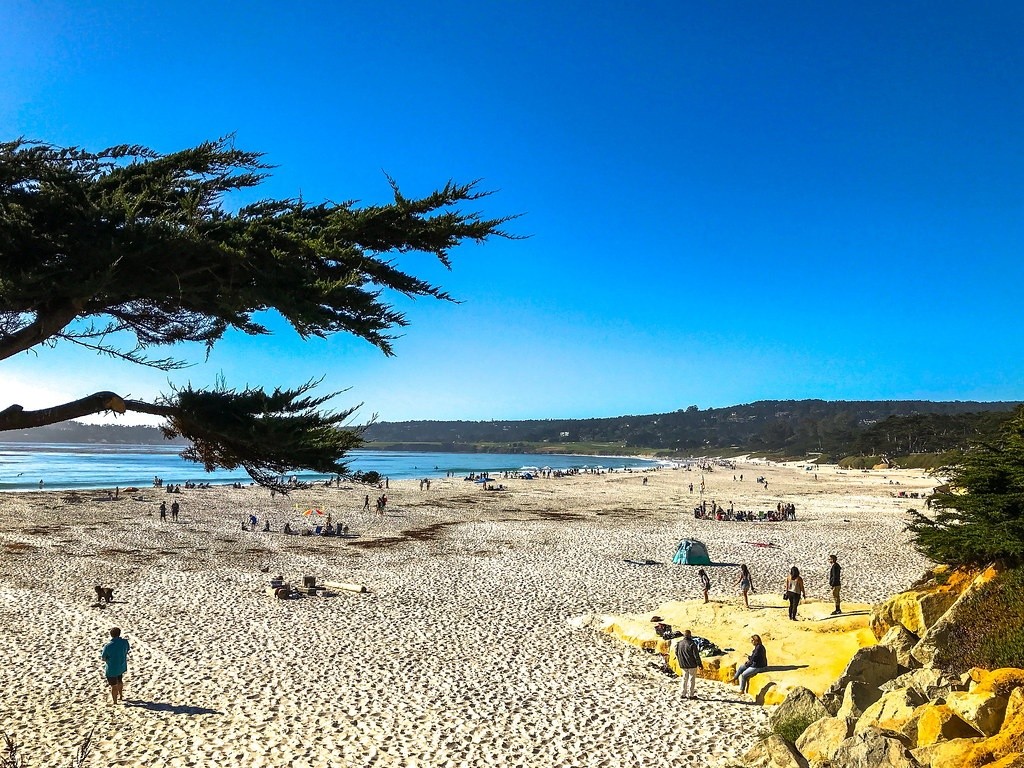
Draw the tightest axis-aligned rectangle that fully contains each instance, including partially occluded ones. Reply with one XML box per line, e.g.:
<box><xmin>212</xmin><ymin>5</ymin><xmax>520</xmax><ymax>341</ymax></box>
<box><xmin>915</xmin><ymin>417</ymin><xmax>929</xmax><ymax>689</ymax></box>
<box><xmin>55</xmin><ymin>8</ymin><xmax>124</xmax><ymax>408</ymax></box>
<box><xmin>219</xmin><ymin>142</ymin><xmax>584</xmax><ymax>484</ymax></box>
<box><xmin>95</xmin><ymin>585</ymin><xmax>114</xmax><ymax>603</ymax></box>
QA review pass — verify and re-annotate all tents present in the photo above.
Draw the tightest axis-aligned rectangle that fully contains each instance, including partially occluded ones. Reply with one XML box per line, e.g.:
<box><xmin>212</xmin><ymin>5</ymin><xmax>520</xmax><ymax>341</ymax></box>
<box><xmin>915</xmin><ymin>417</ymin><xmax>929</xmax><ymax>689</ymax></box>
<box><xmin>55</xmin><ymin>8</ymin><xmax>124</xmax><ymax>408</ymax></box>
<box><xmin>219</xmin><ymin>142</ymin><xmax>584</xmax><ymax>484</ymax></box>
<box><xmin>521</xmin><ymin>467</ymin><xmax>537</xmax><ymax>473</ymax></box>
<box><xmin>672</xmin><ymin>538</ymin><xmax>710</xmax><ymax>565</ymax></box>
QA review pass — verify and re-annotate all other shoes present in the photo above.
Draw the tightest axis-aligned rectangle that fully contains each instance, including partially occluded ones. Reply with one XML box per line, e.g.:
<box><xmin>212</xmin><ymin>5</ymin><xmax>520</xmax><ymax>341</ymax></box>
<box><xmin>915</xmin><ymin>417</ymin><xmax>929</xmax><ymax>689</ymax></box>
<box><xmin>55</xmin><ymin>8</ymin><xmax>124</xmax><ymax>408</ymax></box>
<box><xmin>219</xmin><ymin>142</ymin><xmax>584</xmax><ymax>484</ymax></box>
<box><xmin>681</xmin><ymin>695</ymin><xmax>697</xmax><ymax>700</ymax></box>
<box><xmin>831</xmin><ymin>610</ymin><xmax>842</xmax><ymax>615</ymax></box>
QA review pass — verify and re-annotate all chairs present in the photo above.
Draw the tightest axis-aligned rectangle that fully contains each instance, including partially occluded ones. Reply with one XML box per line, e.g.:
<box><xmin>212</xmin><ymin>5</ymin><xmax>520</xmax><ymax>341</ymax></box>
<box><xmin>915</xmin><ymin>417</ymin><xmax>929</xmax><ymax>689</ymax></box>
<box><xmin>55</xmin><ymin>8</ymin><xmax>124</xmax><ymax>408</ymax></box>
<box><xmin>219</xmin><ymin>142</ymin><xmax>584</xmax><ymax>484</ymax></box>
<box><xmin>316</xmin><ymin>526</ymin><xmax>322</xmax><ymax>535</ymax></box>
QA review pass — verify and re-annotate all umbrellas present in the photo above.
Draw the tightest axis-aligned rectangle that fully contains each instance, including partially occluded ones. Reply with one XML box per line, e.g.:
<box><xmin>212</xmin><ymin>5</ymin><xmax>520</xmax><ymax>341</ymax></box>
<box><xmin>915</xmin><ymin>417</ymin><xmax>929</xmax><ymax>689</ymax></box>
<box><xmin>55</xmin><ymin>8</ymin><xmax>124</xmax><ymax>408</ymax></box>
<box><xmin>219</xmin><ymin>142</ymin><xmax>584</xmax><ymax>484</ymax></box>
<box><xmin>542</xmin><ymin>466</ymin><xmax>551</xmax><ymax>470</ymax></box>
<box><xmin>302</xmin><ymin>509</ymin><xmax>325</xmax><ymax>515</ymax></box>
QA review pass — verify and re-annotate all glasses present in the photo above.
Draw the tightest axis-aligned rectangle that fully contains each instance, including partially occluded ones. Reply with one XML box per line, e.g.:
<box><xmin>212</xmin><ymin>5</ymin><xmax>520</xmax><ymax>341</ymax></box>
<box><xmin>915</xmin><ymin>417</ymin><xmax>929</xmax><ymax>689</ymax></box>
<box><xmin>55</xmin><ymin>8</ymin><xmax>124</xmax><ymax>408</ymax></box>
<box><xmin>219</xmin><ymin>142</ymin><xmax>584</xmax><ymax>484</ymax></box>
<box><xmin>829</xmin><ymin>559</ymin><xmax>830</xmax><ymax>560</ymax></box>
<box><xmin>752</xmin><ymin>640</ymin><xmax>753</xmax><ymax>641</ymax></box>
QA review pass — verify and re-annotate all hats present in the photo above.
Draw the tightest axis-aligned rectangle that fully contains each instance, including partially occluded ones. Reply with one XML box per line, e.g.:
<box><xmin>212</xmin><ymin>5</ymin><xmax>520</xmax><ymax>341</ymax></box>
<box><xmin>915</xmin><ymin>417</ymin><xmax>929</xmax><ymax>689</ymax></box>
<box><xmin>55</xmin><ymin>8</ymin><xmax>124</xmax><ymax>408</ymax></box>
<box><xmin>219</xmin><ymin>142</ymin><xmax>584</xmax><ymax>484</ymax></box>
<box><xmin>698</xmin><ymin>569</ymin><xmax>704</xmax><ymax>575</ymax></box>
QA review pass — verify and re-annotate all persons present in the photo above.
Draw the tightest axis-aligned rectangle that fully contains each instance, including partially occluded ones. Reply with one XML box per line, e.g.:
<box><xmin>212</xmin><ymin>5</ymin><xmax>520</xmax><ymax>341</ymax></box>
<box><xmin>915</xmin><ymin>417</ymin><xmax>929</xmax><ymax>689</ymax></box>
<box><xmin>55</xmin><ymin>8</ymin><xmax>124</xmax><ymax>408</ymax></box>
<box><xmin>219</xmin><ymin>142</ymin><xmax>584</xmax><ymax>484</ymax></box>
<box><xmin>40</xmin><ymin>466</ymin><xmax>841</xmax><ymax>700</ymax></box>
<box><xmin>101</xmin><ymin>627</ymin><xmax>130</xmax><ymax>706</ymax></box>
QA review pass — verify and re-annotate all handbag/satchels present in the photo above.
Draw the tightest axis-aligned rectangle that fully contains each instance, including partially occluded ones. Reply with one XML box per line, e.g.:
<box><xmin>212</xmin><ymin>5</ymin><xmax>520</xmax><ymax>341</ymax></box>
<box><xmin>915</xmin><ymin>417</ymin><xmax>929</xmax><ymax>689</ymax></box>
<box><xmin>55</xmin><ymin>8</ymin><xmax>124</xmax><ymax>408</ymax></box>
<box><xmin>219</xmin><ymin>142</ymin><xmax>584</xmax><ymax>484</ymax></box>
<box><xmin>783</xmin><ymin>594</ymin><xmax>788</xmax><ymax>600</ymax></box>
